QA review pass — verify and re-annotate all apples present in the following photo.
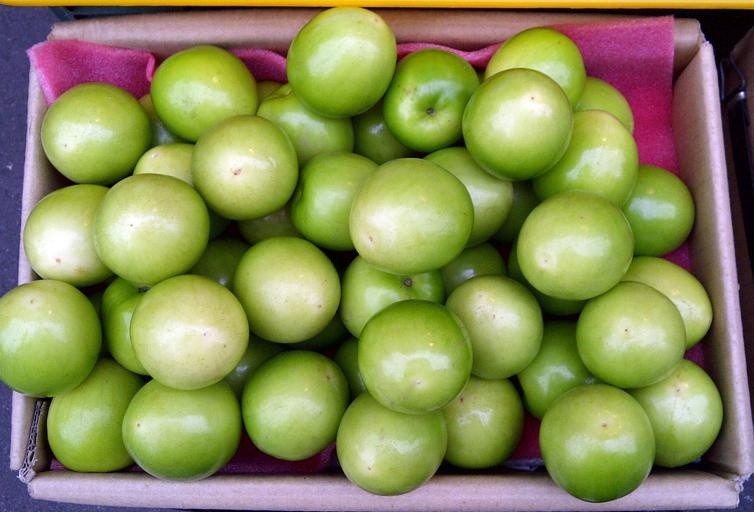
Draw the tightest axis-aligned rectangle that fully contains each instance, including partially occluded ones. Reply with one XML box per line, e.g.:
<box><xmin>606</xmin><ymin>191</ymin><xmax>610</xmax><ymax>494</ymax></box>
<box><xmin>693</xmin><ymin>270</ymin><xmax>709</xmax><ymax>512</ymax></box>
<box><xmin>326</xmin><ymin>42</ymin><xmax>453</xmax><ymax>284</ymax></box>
<box><xmin>1</xmin><ymin>5</ymin><xmax>724</xmax><ymax>503</ymax></box>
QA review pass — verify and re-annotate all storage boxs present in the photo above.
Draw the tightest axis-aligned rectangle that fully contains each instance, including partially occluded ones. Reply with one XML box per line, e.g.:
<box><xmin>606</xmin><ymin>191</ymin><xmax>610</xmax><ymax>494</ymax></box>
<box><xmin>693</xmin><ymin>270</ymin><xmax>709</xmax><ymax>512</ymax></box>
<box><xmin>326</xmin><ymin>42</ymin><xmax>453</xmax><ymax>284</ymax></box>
<box><xmin>10</xmin><ymin>7</ymin><xmax>754</xmax><ymax>512</ymax></box>
